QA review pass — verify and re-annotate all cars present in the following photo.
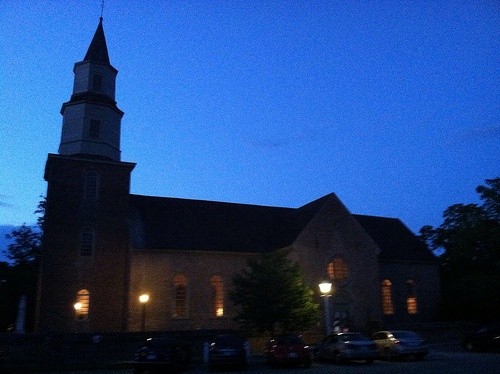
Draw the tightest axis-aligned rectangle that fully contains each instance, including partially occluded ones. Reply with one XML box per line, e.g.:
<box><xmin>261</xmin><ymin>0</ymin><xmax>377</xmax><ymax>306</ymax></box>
<box><xmin>133</xmin><ymin>337</ymin><xmax>192</xmax><ymax>373</ymax></box>
<box><xmin>320</xmin><ymin>333</ymin><xmax>379</xmax><ymax>365</ymax></box>
<box><xmin>372</xmin><ymin>330</ymin><xmax>430</xmax><ymax>361</ymax></box>
<box><xmin>203</xmin><ymin>334</ymin><xmax>251</xmax><ymax>371</ymax></box>
<box><xmin>265</xmin><ymin>334</ymin><xmax>312</xmax><ymax>368</ymax></box>
<box><xmin>461</xmin><ymin>324</ymin><xmax>499</xmax><ymax>353</ymax></box>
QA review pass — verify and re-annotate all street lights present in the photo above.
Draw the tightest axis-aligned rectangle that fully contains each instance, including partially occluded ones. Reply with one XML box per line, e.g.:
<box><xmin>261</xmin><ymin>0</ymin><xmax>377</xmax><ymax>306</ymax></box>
<box><xmin>317</xmin><ymin>274</ymin><xmax>333</xmax><ymax>334</ymax></box>
<box><xmin>139</xmin><ymin>293</ymin><xmax>149</xmax><ymax>340</ymax></box>
